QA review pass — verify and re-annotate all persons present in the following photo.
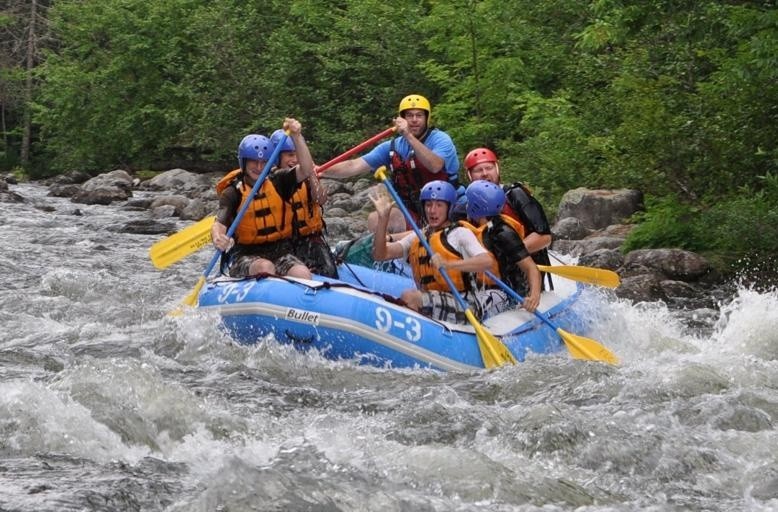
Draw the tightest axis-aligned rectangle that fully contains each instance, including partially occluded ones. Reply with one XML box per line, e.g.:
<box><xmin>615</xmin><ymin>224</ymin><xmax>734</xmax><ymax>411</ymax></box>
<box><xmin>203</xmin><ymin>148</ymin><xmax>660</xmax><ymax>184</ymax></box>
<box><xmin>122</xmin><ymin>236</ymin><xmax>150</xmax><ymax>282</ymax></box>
<box><xmin>313</xmin><ymin>94</ymin><xmax>465</xmax><ymax>233</ymax></box>
<box><xmin>272</xmin><ymin>129</ymin><xmax>338</xmax><ymax>279</ymax></box>
<box><xmin>465</xmin><ymin>148</ymin><xmax>552</xmax><ymax>296</ymax></box>
<box><xmin>368</xmin><ymin>180</ymin><xmax>492</xmax><ymax>324</ymax></box>
<box><xmin>212</xmin><ymin>118</ymin><xmax>314</xmax><ymax>280</ymax></box>
<box><xmin>458</xmin><ymin>181</ymin><xmax>540</xmax><ymax>321</ymax></box>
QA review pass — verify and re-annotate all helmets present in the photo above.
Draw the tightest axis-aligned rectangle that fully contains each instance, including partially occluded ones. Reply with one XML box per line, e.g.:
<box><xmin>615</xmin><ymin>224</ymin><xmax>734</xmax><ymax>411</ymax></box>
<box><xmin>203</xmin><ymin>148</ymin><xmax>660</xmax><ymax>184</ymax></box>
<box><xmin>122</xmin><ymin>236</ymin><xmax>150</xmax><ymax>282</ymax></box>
<box><xmin>238</xmin><ymin>129</ymin><xmax>296</xmax><ymax>171</ymax></box>
<box><xmin>399</xmin><ymin>94</ymin><xmax>431</xmax><ymax>127</ymax></box>
<box><xmin>420</xmin><ymin>148</ymin><xmax>505</xmax><ymax>217</ymax></box>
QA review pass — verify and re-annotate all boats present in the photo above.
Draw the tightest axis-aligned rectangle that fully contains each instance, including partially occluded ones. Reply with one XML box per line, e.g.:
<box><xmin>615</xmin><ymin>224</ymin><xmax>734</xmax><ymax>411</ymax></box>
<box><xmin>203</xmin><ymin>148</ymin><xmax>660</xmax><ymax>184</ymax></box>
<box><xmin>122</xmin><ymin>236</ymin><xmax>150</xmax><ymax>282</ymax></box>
<box><xmin>193</xmin><ymin>235</ymin><xmax>599</xmax><ymax>380</ymax></box>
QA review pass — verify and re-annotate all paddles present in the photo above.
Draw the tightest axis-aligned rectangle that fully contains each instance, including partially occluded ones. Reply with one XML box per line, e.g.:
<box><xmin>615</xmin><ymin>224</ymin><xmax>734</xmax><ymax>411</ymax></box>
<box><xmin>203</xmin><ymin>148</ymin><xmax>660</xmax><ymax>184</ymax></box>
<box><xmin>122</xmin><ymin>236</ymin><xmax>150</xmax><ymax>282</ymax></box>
<box><xmin>374</xmin><ymin>166</ymin><xmax>519</xmax><ymax>368</ymax></box>
<box><xmin>535</xmin><ymin>264</ymin><xmax>623</xmax><ymax>288</ymax></box>
<box><xmin>482</xmin><ymin>268</ymin><xmax>618</xmax><ymax>364</ymax></box>
<box><xmin>165</xmin><ymin>128</ymin><xmax>291</xmax><ymax>316</ymax></box>
<box><xmin>149</xmin><ymin>127</ymin><xmax>396</xmax><ymax>269</ymax></box>
